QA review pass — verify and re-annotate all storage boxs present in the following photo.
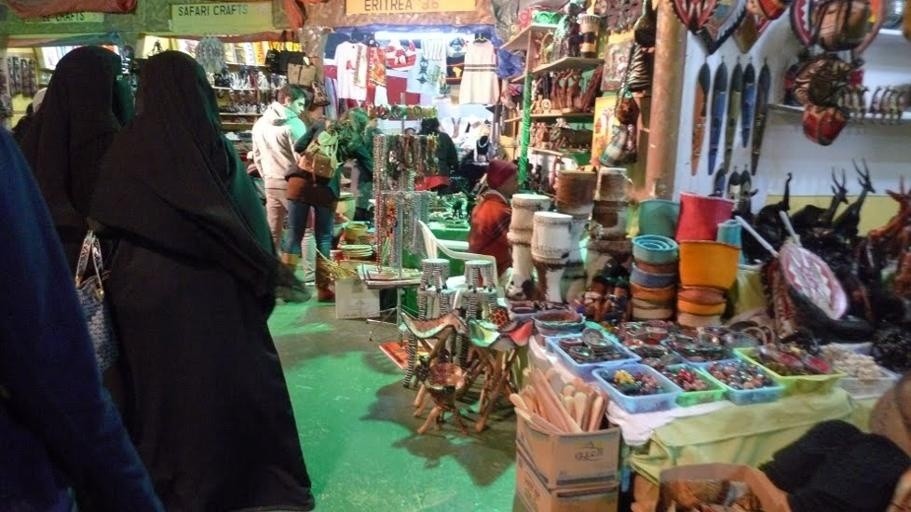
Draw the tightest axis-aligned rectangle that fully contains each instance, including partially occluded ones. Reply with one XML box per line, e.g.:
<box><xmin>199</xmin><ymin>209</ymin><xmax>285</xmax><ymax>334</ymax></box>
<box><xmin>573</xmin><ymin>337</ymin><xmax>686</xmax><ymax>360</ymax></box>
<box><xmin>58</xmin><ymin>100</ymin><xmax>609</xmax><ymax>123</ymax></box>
<box><xmin>519</xmin><ymin>412</ymin><xmax>620</xmax><ymax>490</ymax></box>
<box><xmin>336</xmin><ymin>263</ymin><xmax>380</xmax><ymax>320</ymax></box>
<box><xmin>517</xmin><ymin>447</ymin><xmax>620</xmax><ymax>512</ymax></box>
<box><xmin>513</xmin><ymin>496</ymin><xmax>526</xmax><ymax>512</ymax></box>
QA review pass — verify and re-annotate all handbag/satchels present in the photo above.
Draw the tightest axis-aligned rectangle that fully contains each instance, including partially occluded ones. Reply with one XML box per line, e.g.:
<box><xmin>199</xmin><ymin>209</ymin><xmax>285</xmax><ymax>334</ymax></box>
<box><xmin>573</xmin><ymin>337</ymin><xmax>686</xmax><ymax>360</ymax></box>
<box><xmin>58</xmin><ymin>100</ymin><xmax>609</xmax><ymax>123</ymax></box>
<box><xmin>264</xmin><ymin>48</ymin><xmax>331</xmax><ymax>107</ymax></box>
<box><xmin>615</xmin><ymin>86</ymin><xmax>639</xmax><ymax>124</ymax></box>
<box><xmin>73</xmin><ymin>233</ymin><xmax>120</xmax><ymax>373</ymax></box>
<box><xmin>633</xmin><ymin>1</ymin><xmax>656</xmax><ymax>48</ymax></box>
<box><xmin>793</xmin><ymin>0</ymin><xmax>867</xmax><ymax>146</ymax></box>
<box><xmin>600</xmin><ymin>125</ymin><xmax>638</xmax><ymax>167</ymax></box>
<box><xmin>628</xmin><ymin>47</ymin><xmax>651</xmax><ymax>91</ymax></box>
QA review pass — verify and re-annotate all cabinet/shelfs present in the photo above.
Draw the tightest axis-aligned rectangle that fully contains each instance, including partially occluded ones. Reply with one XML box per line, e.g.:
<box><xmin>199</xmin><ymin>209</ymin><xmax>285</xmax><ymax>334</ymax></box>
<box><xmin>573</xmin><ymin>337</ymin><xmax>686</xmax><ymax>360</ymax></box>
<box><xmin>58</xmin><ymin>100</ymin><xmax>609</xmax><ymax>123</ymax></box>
<box><xmin>500</xmin><ymin>24</ymin><xmax>604</xmax><ymax>200</ymax></box>
<box><xmin>211</xmin><ymin>62</ymin><xmax>274</xmax><ymax>131</ymax></box>
<box><xmin>814</xmin><ymin>20</ymin><xmax>910</xmax><ymax>124</ymax></box>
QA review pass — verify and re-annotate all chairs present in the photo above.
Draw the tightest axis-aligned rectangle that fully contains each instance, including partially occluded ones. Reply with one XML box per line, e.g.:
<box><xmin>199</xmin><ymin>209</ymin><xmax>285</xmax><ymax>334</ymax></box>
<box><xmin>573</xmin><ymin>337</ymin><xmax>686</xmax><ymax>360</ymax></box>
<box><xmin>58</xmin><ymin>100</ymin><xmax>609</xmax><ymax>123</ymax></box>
<box><xmin>418</xmin><ymin>222</ymin><xmax>499</xmax><ymax>297</ymax></box>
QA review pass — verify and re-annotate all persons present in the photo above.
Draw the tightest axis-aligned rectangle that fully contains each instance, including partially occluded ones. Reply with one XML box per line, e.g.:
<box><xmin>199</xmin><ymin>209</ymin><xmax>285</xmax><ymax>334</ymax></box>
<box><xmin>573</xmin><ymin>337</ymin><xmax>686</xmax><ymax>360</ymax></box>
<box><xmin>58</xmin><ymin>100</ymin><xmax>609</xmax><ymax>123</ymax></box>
<box><xmin>75</xmin><ymin>49</ymin><xmax>315</xmax><ymax>511</ymax></box>
<box><xmin>0</xmin><ymin>126</ymin><xmax>164</xmax><ymax>512</ymax></box>
<box><xmin>417</xmin><ymin>117</ymin><xmax>459</xmax><ymax>178</ymax></box>
<box><xmin>280</xmin><ymin>107</ymin><xmax>376</xmax><ymax>302</ymax></box>
<box><xmin>468</xmin><ymin>159</ymin><xmax>520</xmax><ymax>275</ymax></box>
<box><xmin>252</xmin><ymin>84</ymin><xmax>316</xmax><ymax>285</ymax></box>
<box><xmin>11</xmin><ymin>44</ymin><xmax>134</xmax><ymax>287</ymax></box>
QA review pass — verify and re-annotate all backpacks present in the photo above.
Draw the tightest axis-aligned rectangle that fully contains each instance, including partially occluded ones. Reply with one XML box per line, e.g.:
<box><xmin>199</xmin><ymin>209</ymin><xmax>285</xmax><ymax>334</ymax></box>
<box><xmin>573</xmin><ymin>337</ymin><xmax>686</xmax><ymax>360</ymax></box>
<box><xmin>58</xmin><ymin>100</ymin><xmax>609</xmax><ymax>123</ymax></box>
<box><xmin>298</xmin><ymin>121</ymin><xmax>343</xmax><ymax>179</ymax></box>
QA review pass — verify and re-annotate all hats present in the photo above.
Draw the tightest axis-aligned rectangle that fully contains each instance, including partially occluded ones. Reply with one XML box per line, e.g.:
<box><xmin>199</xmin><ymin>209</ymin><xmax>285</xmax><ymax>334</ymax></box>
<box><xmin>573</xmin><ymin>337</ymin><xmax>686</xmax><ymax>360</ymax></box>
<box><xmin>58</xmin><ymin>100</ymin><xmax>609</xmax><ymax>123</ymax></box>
<box><xmin>759</xmin><ymin>419</ymin><xmax>911</xmax><ymax>511</ymax></box>
<box><xmin>489</xmin><ymin>160</ymin><xmax>518</xmax><ymax>188</ymax></box>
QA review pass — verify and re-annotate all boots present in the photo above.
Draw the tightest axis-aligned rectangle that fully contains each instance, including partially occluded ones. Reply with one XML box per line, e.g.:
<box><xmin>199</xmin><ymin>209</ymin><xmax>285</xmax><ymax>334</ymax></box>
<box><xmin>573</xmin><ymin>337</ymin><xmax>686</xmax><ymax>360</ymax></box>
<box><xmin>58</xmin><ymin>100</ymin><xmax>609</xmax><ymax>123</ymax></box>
<box><xmin>315</xmin><ymin>257</ymin><xmax>334</xmax><ymax>302</ymax></box>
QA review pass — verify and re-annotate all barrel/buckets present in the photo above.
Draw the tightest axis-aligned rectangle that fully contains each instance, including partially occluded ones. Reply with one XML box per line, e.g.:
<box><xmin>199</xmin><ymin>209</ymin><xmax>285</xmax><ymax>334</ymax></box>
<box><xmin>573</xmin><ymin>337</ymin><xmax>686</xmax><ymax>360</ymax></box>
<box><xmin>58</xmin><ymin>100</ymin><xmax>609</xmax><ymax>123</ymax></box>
<box><xmin>637</xmin><ymin>192</ymin><xmax>742</xmax><ymax>291</ymax></box>
